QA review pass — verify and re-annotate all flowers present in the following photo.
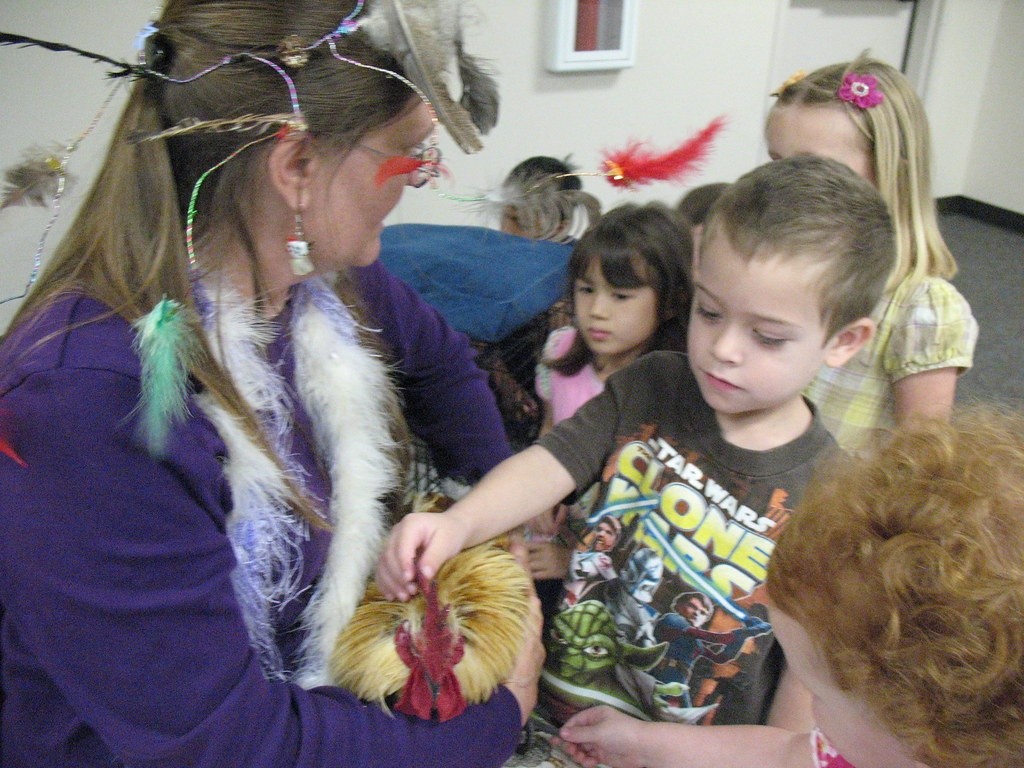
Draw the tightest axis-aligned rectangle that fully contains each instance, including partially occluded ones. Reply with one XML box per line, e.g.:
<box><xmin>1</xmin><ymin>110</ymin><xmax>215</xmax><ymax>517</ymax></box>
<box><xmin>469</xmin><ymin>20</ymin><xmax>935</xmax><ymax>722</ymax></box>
<box><xmin>838</xmin><ymin>72</ymin><xmax>885</xmax><ymax>108</ymax></box>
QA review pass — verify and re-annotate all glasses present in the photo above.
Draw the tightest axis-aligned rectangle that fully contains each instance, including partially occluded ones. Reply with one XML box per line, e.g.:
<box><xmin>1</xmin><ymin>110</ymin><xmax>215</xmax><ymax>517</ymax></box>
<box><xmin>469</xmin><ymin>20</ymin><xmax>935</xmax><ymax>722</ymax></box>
<box><xmin>265</xmin><ymin>128</ymin><xmax>442</xmax><ymax>189</ymax></box>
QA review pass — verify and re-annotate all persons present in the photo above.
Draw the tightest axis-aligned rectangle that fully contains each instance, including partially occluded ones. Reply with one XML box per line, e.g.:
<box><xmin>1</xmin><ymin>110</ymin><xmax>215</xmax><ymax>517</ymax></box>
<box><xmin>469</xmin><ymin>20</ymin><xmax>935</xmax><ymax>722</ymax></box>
<box><xmin>371</xmin><ymin>55</ymin><xmax>1024</xmax><ymax>768</ymax></box>
<box><xmin>0</xmin><ymin>0</ymin><xmax>543</xmax><ymax>767</ymax></box>
